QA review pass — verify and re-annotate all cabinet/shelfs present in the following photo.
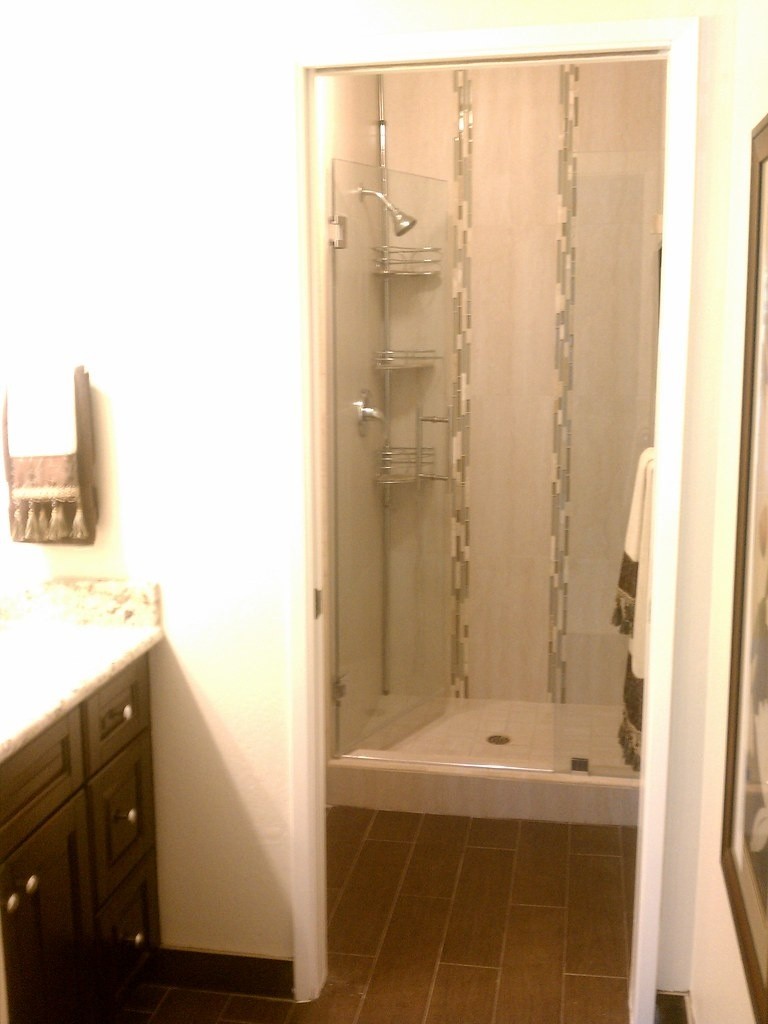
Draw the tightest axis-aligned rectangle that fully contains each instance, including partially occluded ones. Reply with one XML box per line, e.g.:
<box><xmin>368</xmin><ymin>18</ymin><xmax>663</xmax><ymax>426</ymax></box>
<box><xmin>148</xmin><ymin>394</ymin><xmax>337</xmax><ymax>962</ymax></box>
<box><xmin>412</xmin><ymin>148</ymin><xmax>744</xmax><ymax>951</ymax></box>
<box><xmin>1</xmin><ymin>648</ymin><xmax>165</xmax><ymax>1023</ymax></box>
<box><xmin>374</xmin><ymin>243</ymin><xmax>457</xmax><ymax>509</ymax></box>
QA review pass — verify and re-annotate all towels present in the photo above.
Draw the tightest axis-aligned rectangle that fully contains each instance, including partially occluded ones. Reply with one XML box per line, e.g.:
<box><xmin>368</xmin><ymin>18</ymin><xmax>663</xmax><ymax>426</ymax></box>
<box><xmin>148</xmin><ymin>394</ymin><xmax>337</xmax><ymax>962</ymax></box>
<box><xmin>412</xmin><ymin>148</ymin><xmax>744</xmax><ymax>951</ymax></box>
<box><xmin>611</xmin><ymin>444</ymin><xmax>661</xmax><ymax>777</ymax></box>
<box><xmin>3</xmin><ymin>362</ymin><xmax>106</xmax><ymax>553</ymax></box>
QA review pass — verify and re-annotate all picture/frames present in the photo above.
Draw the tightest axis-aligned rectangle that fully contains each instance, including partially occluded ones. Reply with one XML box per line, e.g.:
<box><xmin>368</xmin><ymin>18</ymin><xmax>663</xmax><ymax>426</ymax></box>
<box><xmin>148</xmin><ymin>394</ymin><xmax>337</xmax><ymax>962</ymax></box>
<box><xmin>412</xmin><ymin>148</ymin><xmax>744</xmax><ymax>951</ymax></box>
<box><xmin>717</xmin><ymin>111</ymin><xmax>768</xmax><ymax>1022</ymax></box>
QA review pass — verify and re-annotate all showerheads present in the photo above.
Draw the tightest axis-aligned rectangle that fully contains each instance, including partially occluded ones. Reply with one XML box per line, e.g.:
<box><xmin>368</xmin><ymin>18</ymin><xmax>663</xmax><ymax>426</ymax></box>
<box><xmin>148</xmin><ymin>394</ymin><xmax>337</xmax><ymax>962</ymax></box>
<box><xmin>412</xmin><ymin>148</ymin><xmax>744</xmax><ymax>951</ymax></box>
<box><xmin>392</xmin><ymin>205</ymin><xmax>419</xmax><ymax>239</ymax></box>
